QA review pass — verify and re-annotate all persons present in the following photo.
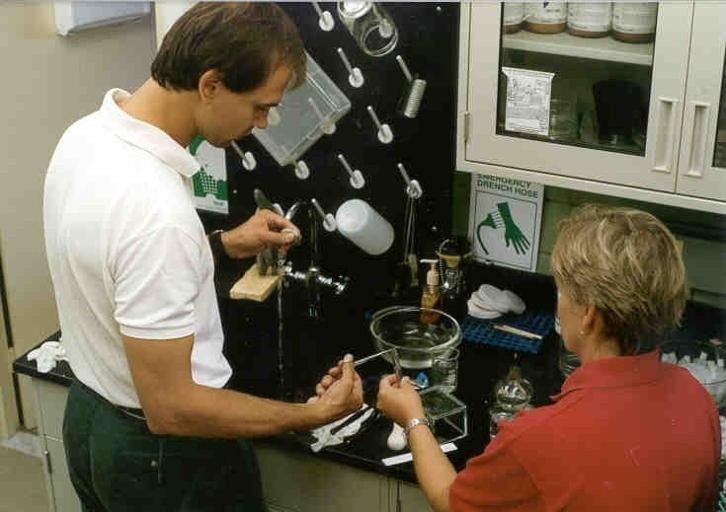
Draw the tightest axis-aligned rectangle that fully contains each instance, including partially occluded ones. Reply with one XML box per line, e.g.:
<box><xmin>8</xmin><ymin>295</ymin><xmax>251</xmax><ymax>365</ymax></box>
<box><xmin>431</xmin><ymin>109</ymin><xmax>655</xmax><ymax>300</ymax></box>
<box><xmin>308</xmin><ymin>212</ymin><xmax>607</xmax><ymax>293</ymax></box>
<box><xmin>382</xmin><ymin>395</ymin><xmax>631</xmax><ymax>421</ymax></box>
<box><xmin>373</xmin><ymin>204</ymin><xmax>722</xmax><ymax>511</ymax></box>
<box><xmin>43</xmin><ymin>0</ymin><xmax>366</xmax><ymax>512</ymax></box>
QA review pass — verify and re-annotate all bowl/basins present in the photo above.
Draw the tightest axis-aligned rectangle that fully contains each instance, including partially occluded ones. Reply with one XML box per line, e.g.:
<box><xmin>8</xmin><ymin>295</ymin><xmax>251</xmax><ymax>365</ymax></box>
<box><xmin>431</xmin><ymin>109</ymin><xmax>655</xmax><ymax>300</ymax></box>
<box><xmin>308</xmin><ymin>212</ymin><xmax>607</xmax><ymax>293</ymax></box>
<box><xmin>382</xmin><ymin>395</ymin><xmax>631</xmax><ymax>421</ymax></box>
<box><xmin>368</xmin><ymin>304</ymin><xmax>465</xmax><ymax>369</ymax></box>
<box><xmin>656</xmin><ymin>339</ymin><xmax>726</xmax><ymax>420</ymax></box>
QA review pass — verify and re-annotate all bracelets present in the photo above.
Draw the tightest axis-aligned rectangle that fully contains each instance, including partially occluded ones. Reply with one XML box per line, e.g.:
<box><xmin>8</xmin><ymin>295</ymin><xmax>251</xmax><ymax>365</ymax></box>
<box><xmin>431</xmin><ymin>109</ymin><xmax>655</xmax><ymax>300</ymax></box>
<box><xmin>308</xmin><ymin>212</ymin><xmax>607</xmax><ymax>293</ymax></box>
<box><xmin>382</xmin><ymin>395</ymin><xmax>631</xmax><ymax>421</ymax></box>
<box><xmin>403</xmin><ymin>415</ymin><xmax>432</xmax><ymax>442</ymax></box>
<box><xmin>209</xmin><ymin>229</ymin><xmax>227</xmax><ymax>262</ymax></box>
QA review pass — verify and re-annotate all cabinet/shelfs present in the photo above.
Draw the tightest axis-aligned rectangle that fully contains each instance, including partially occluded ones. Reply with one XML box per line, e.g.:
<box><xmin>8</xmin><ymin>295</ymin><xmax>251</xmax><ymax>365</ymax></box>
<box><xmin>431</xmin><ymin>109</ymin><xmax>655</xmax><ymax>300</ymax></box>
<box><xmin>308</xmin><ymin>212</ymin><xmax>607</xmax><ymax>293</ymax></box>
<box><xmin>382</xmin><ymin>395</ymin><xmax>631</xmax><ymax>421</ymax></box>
<box><xmin>454</xmin><ymin>2</ymin><xmax>726</xmax><ymax>216</ymax></box>
<box><xmin>388</xmin><ymin>256</ymin><xmax>559</xmax><ymax>510</ymax></box>
<box><xmin>10</xmin><ymin>330</ymin><xmax>86</xmax><ymax>512</ymax></box>
<box><xmin>246</xmin><ymin>300</ymin><xmax>397</xmax><ymax>511</ymax></box>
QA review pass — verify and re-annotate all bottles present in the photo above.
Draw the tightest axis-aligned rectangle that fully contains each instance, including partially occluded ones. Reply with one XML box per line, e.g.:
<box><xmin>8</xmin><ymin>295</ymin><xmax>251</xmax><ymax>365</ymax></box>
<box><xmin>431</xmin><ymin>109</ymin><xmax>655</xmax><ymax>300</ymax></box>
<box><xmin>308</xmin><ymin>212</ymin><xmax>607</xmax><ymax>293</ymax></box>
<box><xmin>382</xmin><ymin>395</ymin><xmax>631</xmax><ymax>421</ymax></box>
<box><xmin>491</xmin><ymin>360</ymin><xmax>534</xmax><ymax>437</ymax></box>
<box><xmin>503</xmin><ymin>1</ymin><xmax>658</xmax><ymax>43</ymax></box>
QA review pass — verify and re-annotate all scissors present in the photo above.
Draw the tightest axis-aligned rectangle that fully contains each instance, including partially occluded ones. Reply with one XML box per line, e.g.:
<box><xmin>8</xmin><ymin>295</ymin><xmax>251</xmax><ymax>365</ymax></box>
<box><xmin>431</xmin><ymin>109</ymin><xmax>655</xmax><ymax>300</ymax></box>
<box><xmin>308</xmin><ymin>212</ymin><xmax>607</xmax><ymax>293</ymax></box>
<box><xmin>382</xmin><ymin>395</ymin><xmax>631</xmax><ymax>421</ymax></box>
<box><xmin>339</xmin><ymin>348</ymin><xmax>396</xmax><ymax>374</ymax></box>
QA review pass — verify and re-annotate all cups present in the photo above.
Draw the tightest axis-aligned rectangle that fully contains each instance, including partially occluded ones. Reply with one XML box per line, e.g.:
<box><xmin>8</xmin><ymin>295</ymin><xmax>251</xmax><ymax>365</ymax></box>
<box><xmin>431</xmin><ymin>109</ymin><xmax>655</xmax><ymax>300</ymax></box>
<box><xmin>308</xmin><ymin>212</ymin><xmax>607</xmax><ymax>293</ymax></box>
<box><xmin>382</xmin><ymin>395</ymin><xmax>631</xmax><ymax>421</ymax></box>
<box><xmin>335</xmin><ymin>200</ymin><xmax>393</xmax><ymax>259</ymax></box>
<box><xmin>429</xmin><ymin>346</ymin><xmax>459</xmax><ymax>395</ymax></box>
<box><xmin>334</xmin><ymin>2</ymin><xmax>399</xmax><ymax>58</ymax></box>
